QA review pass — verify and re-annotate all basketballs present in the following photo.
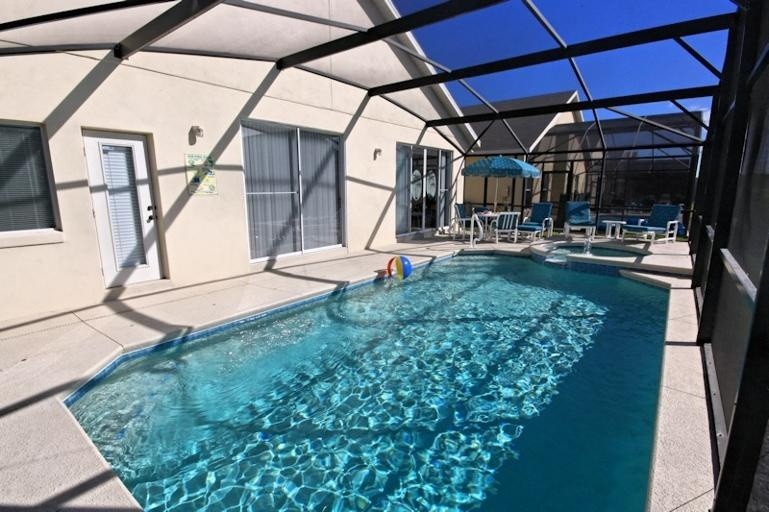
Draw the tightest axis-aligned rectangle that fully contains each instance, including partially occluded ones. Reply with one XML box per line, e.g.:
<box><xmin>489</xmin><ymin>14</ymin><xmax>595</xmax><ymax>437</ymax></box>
<box><xmin>388</xmin><ymin>257</ymin><xmax>410</xmax><ymax>280</ymax></box>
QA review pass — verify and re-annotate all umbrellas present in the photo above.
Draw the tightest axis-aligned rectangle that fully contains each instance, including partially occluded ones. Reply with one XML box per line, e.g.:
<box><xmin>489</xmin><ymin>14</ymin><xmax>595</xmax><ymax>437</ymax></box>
<box><xmin>461</xmin><ymin>154</ymin><xmax>543</xmax><ymax>212</ymax></box>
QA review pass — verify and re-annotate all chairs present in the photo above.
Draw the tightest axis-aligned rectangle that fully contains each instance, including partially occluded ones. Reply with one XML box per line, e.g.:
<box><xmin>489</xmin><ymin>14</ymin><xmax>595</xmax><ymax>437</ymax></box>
<box><xmin>621</xmin><ymin>203</ymin><xmax>681</xmax><ymax>245</ymax></box>
<box><xmin>564</xmin><ymin>200</ymin><xmax>596</xmax><ymax>241</ymax></box>
<box><xmin>450</xmin><ymin>202</ymin><xmax>554</xmax><ymax>240</ymax></box>
